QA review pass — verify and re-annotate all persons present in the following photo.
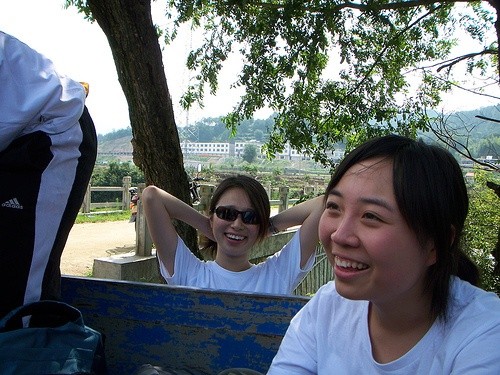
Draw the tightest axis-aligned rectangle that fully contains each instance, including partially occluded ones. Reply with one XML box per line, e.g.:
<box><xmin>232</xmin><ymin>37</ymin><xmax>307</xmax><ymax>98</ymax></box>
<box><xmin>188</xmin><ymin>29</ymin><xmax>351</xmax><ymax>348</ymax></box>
<box><xmin>266</xmin><ymin>135</ymin><xmax>500</xmax><ymax>374</ymax></box>
<box><xmin>0</xmin><ymin>30</ymin><xmax>98</xmax><ymax>333</ymax></box>
<box><xmin>140</xmin><ymin>176</ymin><xmax>328</xmax><ymax>296</ymax></box>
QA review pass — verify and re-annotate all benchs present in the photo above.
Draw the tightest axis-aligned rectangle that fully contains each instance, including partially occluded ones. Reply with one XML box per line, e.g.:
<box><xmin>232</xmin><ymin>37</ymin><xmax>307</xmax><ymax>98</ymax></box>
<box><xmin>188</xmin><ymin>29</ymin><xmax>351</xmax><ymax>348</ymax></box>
<box><xmin>54</xmin><ymin>277</ymin><xmax>312</xmax><ymax>374</ymax></box>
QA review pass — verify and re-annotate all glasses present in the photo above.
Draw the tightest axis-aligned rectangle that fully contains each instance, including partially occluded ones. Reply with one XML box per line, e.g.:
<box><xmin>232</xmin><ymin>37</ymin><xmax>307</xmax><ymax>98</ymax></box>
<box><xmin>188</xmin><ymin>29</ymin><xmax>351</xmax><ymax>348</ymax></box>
<box><xmin>211</xmin><ymin>206</ymin><xmax>262</xmax><ymax>225</ymax></box>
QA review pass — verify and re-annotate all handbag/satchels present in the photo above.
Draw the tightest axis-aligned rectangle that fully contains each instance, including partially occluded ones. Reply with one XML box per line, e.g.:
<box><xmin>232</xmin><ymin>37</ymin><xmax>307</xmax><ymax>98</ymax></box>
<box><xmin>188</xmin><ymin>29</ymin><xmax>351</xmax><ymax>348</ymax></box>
<box><xmin>1</xmin><ymin>301</ymin><xmax>109</xmax><ymax>374</ymax></box>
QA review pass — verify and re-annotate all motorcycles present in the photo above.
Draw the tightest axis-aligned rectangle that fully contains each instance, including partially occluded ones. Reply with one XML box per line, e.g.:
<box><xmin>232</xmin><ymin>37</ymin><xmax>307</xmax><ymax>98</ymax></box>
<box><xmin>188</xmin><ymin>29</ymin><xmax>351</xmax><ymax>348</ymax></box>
<box><xmin>128</xmin><ymin>162</ymin><xmax>205</xmax><ymax>232</ymax></box>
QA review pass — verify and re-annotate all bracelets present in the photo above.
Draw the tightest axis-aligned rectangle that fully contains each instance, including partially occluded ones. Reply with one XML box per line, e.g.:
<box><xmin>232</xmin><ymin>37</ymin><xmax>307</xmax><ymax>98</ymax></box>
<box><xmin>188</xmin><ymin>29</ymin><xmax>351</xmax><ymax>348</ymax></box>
<box><xmin>268</xmin><ymin>218</ymin><xmax>279</xmax><ymax>235</ymax></box>
